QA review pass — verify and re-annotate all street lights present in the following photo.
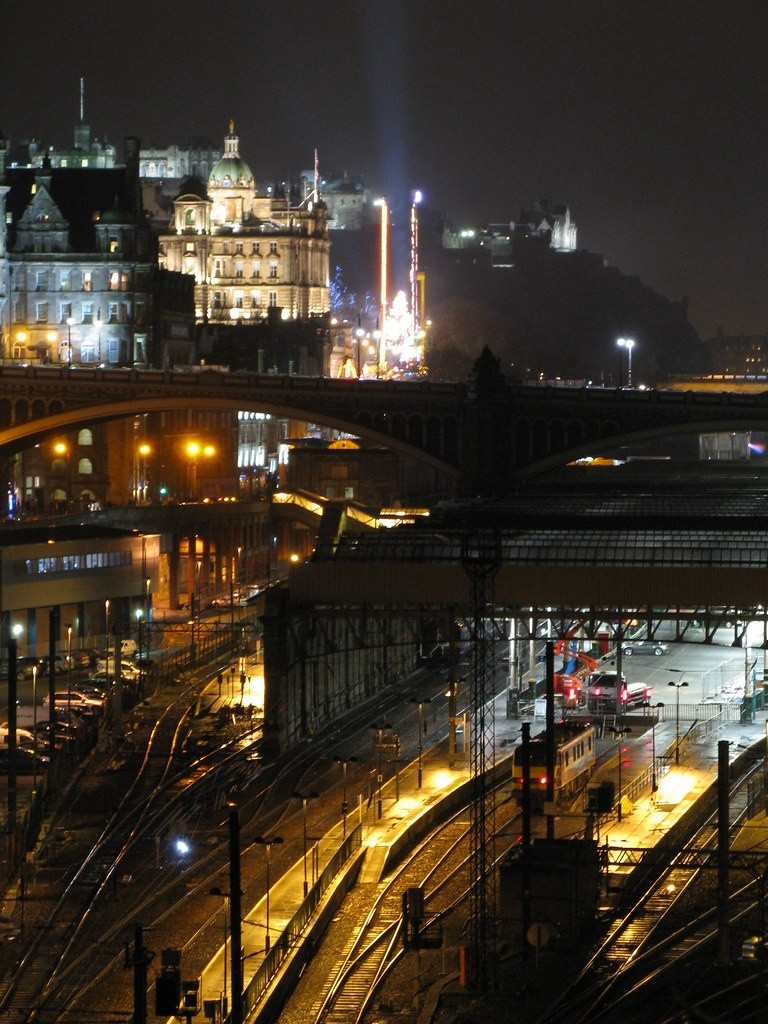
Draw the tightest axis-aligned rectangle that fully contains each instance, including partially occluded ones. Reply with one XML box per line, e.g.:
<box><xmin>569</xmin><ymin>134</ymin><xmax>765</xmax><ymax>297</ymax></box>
<box><xmin>208</xmin><ymin>886</ymin><xmax>247</xmax><ymax>1019</ymax></box>
<box><xmin>92</xmin><ymin>317</ymin><xmax>106</xmax><ymax>368</ymax></box>
<box><xmin>287</xmin><ymin>791</ymin><xmax>322</xmax><ymax>899</ymax></box>
<box><xmin>64</xmin><ymin>316</ymin><xmax>80</xmax><ymax>365</ymax></box>
<box><xmin>138</xmin><ymin>442</ymin><xmax>153</xmax><ymax>506</ymax></box>
<box><xmin>252</xmin><ymin>835</ymin><xmax>286</xmax><ymax>954</ymax></box>
<box><xmin>331</xmin><ymin>754</ymin><xmax>360</xmax><ymax>841</ymax></box>
<box><xmin>369</xmin><ymin>723</ymin><xmax>391</xmax><ymax>819</ymax></box>
<box><xmin>188</xmin><ymin>444</ymin><xmax>214</xmax><ymax>503</ymax></box>
<box><xmin>667</xmin><ymin>680</ymin><xmax>689</xmax><ymax>765</ymax></box>
<box><xmin>617</xmin><ymin>337</ymin><xmax>634</xmax><ymax>391</ymax></box>
<box><xmin>406</xmin><ymin>694</ymin><xmax>433</xmax><ymax>789</ymax></box>
<box><xmin>641</xmin><ymin>702</ymin><xmax>666</xmax><ymax>791</ymax></box>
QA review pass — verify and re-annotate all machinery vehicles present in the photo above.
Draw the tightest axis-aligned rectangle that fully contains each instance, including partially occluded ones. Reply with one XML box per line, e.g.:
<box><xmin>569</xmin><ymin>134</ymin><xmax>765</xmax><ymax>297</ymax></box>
<box><xmin>552</xmin><ymin>618</ymin><xmax>598</xmax><ymax>709</ymax></box>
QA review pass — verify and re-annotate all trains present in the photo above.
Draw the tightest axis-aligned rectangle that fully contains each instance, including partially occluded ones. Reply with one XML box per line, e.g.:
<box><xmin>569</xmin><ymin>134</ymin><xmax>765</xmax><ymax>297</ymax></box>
<box><xmin>511</xmin><ymin>720</ymin><xmax>601</xmax><ymax>813</ymax></box>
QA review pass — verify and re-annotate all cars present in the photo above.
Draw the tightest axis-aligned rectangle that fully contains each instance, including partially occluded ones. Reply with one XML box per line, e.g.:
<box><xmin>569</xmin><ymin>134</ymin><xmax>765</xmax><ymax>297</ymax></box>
<box><xmin>0</xmin><ymin>674</ymin><xmax>150</xmax><ymax>775</ymax></box>
<box><xmin>211</xmin><ymin>585</ymin><xmax>264</xmax><ymax>609</ymax></box>
<box><xmin>107</xmin><ymin>639</ymin><xmax>139</xmax><ymax>657</ymax></box>
<box><xmin>620</xmin><ymin>638</ymin><xmax>670</xmax><ymax>656</ymax></box>
<box><xmin>1</xmin><ymin>647</ymin><xmax>101</xmax><ymax>680</ymax></box>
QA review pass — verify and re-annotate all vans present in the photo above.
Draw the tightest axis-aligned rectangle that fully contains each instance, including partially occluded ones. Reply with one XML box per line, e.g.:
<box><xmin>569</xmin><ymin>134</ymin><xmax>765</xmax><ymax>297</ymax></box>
<box><xmin>586</xmin><ymin>670</ymin><xmax>634</xmax><ymax>715</ymax></box>
<box><xmin>96</xmin><ymin>658</ymin><xmax>150</xmax><ymax>682</ymax></box>
